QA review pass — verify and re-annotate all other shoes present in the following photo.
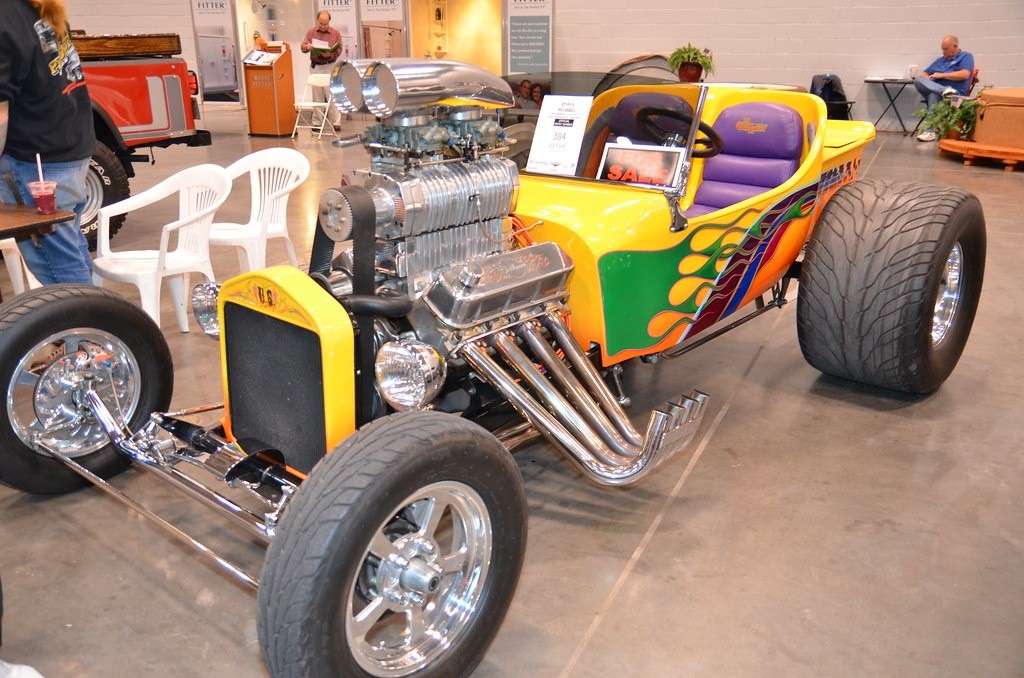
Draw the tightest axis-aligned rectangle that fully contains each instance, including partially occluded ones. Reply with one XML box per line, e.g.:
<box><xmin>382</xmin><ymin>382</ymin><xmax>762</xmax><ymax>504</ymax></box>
<box><xmin>334</xmin><ymin>125</ymin><xmax>341</xmax><ymax>131</ymax></box>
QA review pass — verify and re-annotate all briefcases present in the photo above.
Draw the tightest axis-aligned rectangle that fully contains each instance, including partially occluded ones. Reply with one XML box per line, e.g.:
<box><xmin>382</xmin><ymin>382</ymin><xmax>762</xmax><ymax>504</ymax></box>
<box><xmin>313</xmin><ymin>125</ymin><xmax>321</xmax><ymax>131</ymax></box>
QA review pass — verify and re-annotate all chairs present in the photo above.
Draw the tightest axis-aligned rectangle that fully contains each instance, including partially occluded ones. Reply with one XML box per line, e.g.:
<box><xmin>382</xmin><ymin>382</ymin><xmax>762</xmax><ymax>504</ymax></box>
<box><xmin>606</xmin><ymin>91</ymin><xmax>693</xmax><ymax>146</ymax></box>
<box><xmin>90</xmin><ymin>163</ymin><xmax>233</xmax><ymax>332</ymax></box>
<box><xmin>809</xmin><ymin>74</ymin><xmax>855</xmax><ymax>120</ymax></box>
<box><xmin>291</xmin><ymin>74</ymin><xmax>338</xmax><ymax>139</ymax></box>
<box><xmin>910</xmin><ymin>67</ymin><xmax>979</xmax><ymax>136</ymax></box>
<box><xmin>683</xmin><ymin>102</ymin><xmax>805</xmax><ymax>219</ymax></box>
<box><xmin>208</xmin><ymin>147</ymin><xmax>310</xmax><ymax>273</ymax></box>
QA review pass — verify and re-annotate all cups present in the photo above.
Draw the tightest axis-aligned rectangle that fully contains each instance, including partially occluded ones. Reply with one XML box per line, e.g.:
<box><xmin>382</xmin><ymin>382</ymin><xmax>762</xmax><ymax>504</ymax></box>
<box><xmin>909</xmin><ymin>65</ymin><xmax>918</xmax><ymax>80</ymax></box>
<box><xmin>28</xmin><ymin>182</ymin><xmax>57</xmax><ymax>215</ymax></box>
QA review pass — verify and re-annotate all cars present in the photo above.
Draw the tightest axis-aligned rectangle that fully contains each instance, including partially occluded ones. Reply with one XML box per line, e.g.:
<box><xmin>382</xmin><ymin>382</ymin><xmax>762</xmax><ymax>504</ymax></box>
<box><xmin>69</xmin><ymin>30</ymin><xmax>213</xmax><ymax>257</ymax></box>
<box><xmin>1</xmin><ymin>58</ymin><xmax>988</xmax><ymax>676</ymax></box>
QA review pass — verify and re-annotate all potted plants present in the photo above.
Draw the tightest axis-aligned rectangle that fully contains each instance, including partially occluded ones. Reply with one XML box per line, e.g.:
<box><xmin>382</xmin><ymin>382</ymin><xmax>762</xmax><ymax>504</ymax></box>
<box><xmin>666</xmin><ymin>42</ymin><xmax>716</xmax><ymax>82</ymax></box>
<box><xmin>912</xmin><ymin>99</ymin><xmax>982</xmax><ymax>140</ymax></box>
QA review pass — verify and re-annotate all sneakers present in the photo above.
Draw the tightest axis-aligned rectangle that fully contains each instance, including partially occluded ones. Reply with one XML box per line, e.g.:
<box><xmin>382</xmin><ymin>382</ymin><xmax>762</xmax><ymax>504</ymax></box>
<box><xmin>942</xmin><ymin>86</ymin><xmax>956</xmax><ymax>98</ymax></box>
<box><xmin>917</xmin><ymin>132</ymin><xmax>935</xmax><ymax>141</ymax></box>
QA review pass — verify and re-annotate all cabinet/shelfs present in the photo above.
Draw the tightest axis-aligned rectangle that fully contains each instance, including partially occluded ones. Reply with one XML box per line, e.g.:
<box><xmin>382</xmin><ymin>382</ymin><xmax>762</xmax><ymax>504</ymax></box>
<box><xmin>430</xmin><ymin>0</ymin><xmax>448</xmax><ymax>52</ymax></box>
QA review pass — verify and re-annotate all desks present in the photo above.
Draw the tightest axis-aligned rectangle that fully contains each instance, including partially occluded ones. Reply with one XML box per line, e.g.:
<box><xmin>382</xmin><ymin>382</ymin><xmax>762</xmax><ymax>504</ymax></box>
<box><xmin>0</xmin><ymin>202</ymin><xmax>77</xmax><ymax>296</ymax></box>
<box><xmin>864</xmin><ymin>77</ymin><xmax>915</xmax><ymax>136</ymax></box>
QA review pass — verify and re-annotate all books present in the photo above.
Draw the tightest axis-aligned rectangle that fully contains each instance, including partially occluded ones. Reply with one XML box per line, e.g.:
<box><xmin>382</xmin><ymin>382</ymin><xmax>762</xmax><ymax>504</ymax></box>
<box><xmin>264</xmin><ymin>44</ymin><xmax>286</xmax><ymax>53</ymax></box>
<box><xmin>310</xmin><ymin>41</ymin><xmax>338</xmax><ymax>56</ymax></box>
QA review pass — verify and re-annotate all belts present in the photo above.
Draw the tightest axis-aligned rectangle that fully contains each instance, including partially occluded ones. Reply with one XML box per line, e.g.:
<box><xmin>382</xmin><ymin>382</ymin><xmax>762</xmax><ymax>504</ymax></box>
<box><xmin>312</xmin><ymin>61</ymin><xmax>332</xmax><ymax>65</ymax></box>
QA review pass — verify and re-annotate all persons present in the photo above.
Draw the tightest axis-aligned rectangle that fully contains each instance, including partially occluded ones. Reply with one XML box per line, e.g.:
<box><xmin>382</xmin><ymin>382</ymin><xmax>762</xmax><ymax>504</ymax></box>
<box><xmin>300</xmin><ymin>10</ymin><xmax>341</xmax><ymax>134</ymax></box>
<box><xmin>912</xmin><ymin>35</ymin><xmax>975</xmax><ymax>141</ymax></box>
<box><xmin>514</xmin><ymin>79</ymin><xmax>544</xmax><ymax>109</ymax></box>
<box><xmin>0</xmin><ymin>0</ymin><xmax>99</xmax><ymax>374</ymax></box>
